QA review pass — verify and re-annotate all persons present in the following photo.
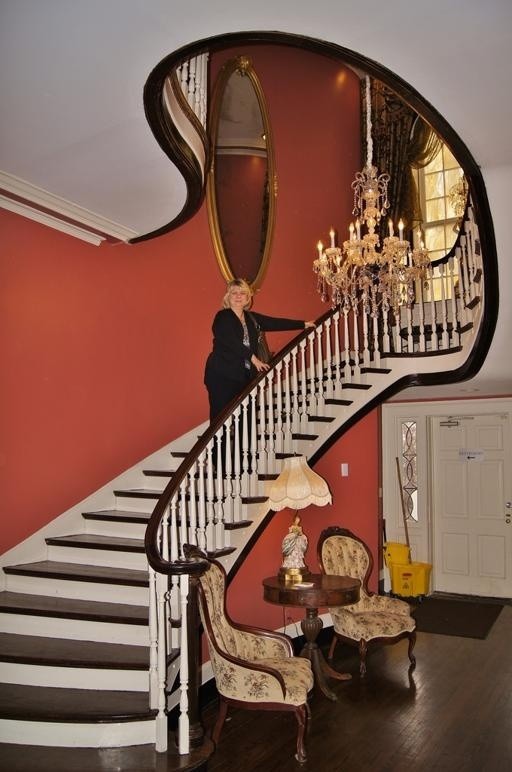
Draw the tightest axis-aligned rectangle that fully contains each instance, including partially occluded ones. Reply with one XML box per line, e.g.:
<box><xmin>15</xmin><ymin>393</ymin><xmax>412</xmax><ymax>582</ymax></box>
<box><xmin>205</xmin><ymin>277</ymin><xmax>317</xmax><ymax>477</ymax></box>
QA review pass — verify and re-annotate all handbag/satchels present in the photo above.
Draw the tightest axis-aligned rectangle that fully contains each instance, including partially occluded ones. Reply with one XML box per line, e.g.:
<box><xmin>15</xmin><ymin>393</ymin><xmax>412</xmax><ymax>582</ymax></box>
<box><xmin>247</xmin><ymin>311</ymin><xmax>271</xmax><ymax>363</ymax></box>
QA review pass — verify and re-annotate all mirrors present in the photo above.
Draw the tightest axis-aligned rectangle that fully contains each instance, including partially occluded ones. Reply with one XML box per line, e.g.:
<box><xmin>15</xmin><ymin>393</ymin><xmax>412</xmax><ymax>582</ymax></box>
<box><xmin>206</xmin><ymin>54</ymin><xmax>277</xmax><ymax>299</ymax></box>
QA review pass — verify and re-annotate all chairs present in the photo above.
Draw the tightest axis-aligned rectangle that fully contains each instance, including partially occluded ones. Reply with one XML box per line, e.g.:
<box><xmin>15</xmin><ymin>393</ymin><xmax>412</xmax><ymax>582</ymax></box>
<box><xmin>178</xmin><ymin>540</ymin><xmax>316</xmax><ymax>763</ymax></box>
<box><xmin>317</xmin><ymin>524</ymin><xmax>420</xmax><ymax>702</ymax></box>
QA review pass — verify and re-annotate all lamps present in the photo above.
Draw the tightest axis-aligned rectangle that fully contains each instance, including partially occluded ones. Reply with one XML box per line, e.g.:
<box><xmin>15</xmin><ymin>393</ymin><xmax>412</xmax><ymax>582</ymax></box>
<box><xmin>270</xmin><ymin>452</ymin><xmax>333</xmax><ymax>590</ymax></box>
<box><xmin>309</xmin><ymin>70</ymin><xmax>429</xmax><ymax>326</ymax></box>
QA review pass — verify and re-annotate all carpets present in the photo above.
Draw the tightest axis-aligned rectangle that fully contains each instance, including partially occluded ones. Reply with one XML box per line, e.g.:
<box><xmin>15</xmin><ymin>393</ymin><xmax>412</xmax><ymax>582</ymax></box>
<box><xmin>408</xmin><ymin>592</ymin><xmax>504</xmax><ymax>641</ymax></box>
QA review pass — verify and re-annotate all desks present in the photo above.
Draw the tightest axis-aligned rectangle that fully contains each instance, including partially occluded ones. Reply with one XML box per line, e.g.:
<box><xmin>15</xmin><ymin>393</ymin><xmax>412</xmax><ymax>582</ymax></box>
<box><xmin>262</xmin><ymin>568</ymin><xmax>361</xmax><ymax>705</ymax></box>
